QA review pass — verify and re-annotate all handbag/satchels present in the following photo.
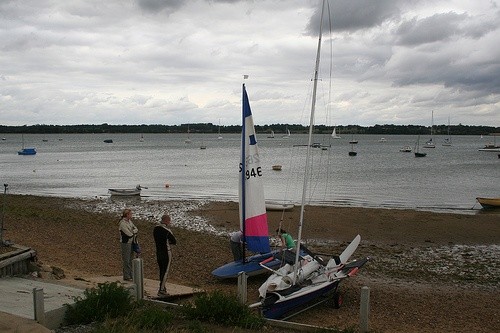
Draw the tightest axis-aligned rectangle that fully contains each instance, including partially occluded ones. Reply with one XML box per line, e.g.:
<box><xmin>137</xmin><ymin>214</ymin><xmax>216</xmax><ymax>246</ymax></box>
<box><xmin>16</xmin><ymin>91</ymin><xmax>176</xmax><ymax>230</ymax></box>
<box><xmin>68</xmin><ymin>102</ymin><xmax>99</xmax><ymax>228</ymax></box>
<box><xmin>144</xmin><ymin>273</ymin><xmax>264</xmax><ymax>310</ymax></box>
<box><xmin>133</xmin><ymin>233</ymin><xmax>141</xmax><ymax>253</ymax></box>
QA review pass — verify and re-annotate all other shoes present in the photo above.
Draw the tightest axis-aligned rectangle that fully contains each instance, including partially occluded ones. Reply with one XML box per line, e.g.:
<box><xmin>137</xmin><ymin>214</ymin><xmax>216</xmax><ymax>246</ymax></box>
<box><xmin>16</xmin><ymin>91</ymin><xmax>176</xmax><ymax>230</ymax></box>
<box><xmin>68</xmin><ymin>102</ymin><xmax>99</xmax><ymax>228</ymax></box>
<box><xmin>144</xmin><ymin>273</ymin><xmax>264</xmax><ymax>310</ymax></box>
<box><xmin>123</xmin><ymin>277</ymin><xmax>133</xmax><ymax>281</ymax></box>
<box><xmin>157</xmin><ymin>287</ymin><xmax>169</xmax><ymax>296</ymax></box>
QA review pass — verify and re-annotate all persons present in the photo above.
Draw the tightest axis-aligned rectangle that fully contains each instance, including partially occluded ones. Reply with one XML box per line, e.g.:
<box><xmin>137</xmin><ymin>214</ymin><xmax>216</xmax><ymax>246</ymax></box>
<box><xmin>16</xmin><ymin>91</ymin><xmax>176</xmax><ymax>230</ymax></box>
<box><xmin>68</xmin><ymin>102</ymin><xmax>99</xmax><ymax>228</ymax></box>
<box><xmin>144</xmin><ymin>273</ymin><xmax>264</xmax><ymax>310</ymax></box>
<box><xmin>231</xmin><ymin>230</ymin><xmax>243</xmax><ymax>260</ymax></box>
<box><xmin>276</xmin><ymin>228</ymin><xmax>296</xmax><ymax>253</ymax></box>
<box><xmin>154</xmin><ymin>214</ymin><xmax>177</xmax><ymax>296</ymax></box>
<box><xmin>118</xmin><ymin>209</ymin><xmax>138</xmax><ymax>282</ymax></box>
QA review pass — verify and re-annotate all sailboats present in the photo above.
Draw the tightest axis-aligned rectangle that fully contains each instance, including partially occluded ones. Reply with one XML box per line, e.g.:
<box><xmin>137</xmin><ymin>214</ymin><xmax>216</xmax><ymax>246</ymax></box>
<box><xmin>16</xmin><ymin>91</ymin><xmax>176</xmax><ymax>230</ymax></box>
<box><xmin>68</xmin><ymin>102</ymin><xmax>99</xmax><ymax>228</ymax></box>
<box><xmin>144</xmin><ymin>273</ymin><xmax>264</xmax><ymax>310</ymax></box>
<box><xmin>423</xmin><ymin>110</ymin><xmax>435</xmax><ymax>148</ymax></box>
<box><xmin>266</xmin><ymin>130</ymin><xmax>274</xmax><ymax>138</ymax></box>
<box><xmin>282</xmin><ymin>125</ymin><xmax>290</xmax><ymax>139</ymax></box>
<box><xmin>185</xmin><ymin>121</ymin><xmax>192</xmax><ymax>143</ymax></box>
<box><xmin>441</xmin><ymin>116</ymin><xmax>452</xmax><ymax>146</ymax></box>
<box><xmin>413</xmin><ymin>135</ymin><xmax>427</xmax><ymax>157</ymax></box>
<box><xmin>331</xmin><ymin>124</ymin><xmax>341</xmax><ymax>139</ymax></box>
<box><xmin>258</xmin><ymin>1</ymin><xmax>371</xmax><ymax>321</ymax></box>
<box><xmin>210</xmin><ymin>83</ymin><xmax>307</xmax><ymax>282</ymax></box>
<box><xmin>17</xmin><ymin>132</ymin><xmax>37</xmax><ymax>155</ymax></box>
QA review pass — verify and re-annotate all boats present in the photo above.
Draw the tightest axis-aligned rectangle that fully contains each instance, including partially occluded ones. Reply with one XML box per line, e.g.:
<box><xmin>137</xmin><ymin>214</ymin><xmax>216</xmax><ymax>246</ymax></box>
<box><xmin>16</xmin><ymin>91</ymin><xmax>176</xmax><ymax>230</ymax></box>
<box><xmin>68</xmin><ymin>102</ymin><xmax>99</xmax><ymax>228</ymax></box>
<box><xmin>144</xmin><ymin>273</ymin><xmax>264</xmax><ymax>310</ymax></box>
<box><xmin>200</xmin><ymin>145</ymin><xmax>206</xmax><ymax>149</ymax></box>
<box><xmin>400</xmin><ymin>145</ymin><xmax>413</xmax><ymax>152</ymax></box>
<box><xmin>476</xmin><ymin>197</ymin><xmax>500</xmax><ymax>209</ymax></box>
<box><xmin>108</xmin><ymin>184</ymin><xmax>148</xmax><ymax>196</ymax></box>
<box><xmin>349</xmin><ymin>143</ymin><xmax>357</xmax><ymax>156</ymax></box>
<box><xmin>349</xmin><ymin>140</ymin><xmax>359</xmax><ymax>144</ymax></box>
<box><xmin>377</xmin><ymin>137</ymin><xmax>388</xmax><ymax>143</ymax></box>
<box><xmin>310</xmin><ymin>142</ymin><xmax>327</xmax><ymax>150</ymax></box>
<box><xmin>272</xmin><ymin>165</ymin><xmax>282</xmax><ymax>170</ymax></box>
<box><xmin>477</xmin><ymin>144</ymin><xmax>500</xmax><ymax>152</ymax></box>
